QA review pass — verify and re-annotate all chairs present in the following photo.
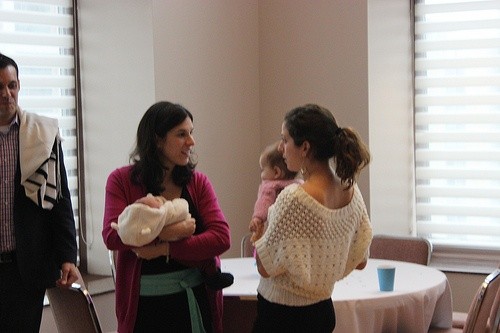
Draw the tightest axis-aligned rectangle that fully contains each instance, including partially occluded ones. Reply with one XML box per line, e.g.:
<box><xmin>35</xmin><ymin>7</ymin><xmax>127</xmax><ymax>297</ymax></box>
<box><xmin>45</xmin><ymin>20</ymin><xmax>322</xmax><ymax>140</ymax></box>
<box><xmin>47</xmin><ymin>234</ymin><xmax>500</xmax><ymax>333</ymax></box>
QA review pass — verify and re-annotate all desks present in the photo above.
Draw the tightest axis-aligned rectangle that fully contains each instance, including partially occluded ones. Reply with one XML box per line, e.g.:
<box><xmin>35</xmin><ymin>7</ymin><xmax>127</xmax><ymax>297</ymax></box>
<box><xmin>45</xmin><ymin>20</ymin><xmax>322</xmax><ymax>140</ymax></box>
<box><xmin>219</xmin><ymin>256</ymin><xmax>453</xmax><ymax>333</ymax></box>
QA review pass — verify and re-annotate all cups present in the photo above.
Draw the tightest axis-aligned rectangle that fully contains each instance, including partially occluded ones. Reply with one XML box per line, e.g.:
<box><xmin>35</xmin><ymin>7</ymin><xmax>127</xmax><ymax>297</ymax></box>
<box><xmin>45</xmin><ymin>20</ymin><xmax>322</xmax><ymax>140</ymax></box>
<box><xmin>376</xmin><ymin>265</ymin><xmax>396</xmax><ymax>291</ymax></box>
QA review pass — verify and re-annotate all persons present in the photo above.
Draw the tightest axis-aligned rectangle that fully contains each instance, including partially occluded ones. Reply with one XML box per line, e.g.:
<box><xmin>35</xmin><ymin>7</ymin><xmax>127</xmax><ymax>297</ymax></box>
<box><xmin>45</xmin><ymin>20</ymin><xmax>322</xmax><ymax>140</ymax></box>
<box><xmin>111</xmin><ymin>193</ymin><xmax>191</xmax><ymax>247</ymax></box>
<box><xmin>249</xmin><ymin>144</ymin><xmax>304</xmax><ymax>234</ymax></box>
<box><xmin>0</xmin><ymin>53</ymin><xmax>77</xmax><ymax>333</ymax></box>
<box><xmin>101</xmin><ymin>102</ymin><xmax>234</xmax><ymax>333</ymax></box>
<box><xmin>254</xmin><ymin>104</ymin><xmax>373</xmax><ymax>333</ymax></box>
